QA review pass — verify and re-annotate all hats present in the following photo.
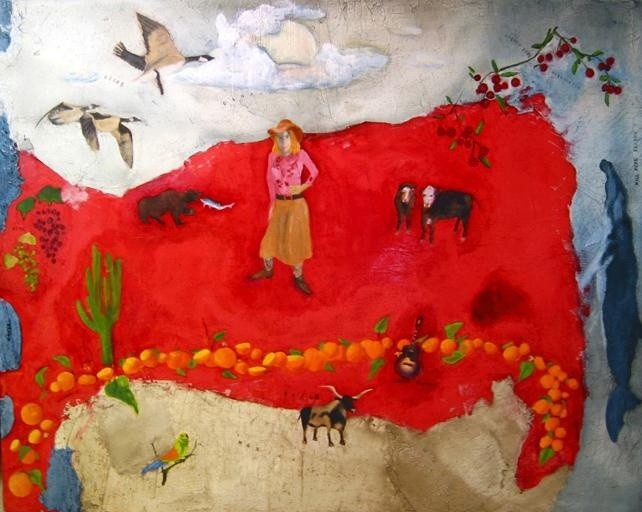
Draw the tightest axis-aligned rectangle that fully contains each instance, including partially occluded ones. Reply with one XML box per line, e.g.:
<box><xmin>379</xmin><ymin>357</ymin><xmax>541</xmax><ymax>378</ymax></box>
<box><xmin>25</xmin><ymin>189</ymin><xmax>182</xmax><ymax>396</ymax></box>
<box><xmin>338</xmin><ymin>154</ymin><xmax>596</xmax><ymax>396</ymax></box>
<box><xmin>268</xmin><ymin>119</ymin><xmax>303</xmax><ymax>142</ymax></box>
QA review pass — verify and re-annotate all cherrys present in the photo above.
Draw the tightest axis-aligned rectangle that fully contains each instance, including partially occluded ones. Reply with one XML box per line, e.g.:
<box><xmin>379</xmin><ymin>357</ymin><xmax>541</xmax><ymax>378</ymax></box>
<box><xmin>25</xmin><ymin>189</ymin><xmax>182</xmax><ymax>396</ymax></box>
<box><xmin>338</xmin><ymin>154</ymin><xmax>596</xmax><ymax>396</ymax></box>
<box><xmin>435</xmin><ymin>37</ymin><xmax>622</xmax><ymax>166</ymax></box>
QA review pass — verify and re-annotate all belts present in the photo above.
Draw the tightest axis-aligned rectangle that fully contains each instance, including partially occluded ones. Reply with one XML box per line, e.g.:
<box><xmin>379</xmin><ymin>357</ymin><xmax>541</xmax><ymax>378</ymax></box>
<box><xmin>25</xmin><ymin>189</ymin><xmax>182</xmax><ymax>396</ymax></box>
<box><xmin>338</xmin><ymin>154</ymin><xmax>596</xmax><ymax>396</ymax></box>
<box><xmin>276</xmin><ymin>194</ymin><xmax>303</xmax><ymax>200</ymax></box>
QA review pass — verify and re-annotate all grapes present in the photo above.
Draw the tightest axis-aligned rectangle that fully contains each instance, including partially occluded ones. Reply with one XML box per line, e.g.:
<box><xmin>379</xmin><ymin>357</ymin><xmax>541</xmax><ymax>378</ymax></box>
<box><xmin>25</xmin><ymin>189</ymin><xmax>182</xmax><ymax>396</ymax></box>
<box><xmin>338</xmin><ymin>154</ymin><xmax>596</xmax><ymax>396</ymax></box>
<box><xmin>11</xmin><ymin>201</ymin><xmax>65</xmax><ymax>294</ymax></box>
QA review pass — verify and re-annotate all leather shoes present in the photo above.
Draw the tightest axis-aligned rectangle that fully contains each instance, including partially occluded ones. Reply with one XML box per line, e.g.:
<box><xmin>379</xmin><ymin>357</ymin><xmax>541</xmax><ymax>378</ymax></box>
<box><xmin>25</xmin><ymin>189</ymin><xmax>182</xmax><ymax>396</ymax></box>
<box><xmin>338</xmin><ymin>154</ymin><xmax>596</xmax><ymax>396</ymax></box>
<box><xmin>296</xmin><ymin>275</ymin><xmax>311</xmax><ymax>294</ymax></box>
<box><xmin>251</xmin><ymin>267</ymin><xmax>273</xmax><ymax>279</ymax></box>
